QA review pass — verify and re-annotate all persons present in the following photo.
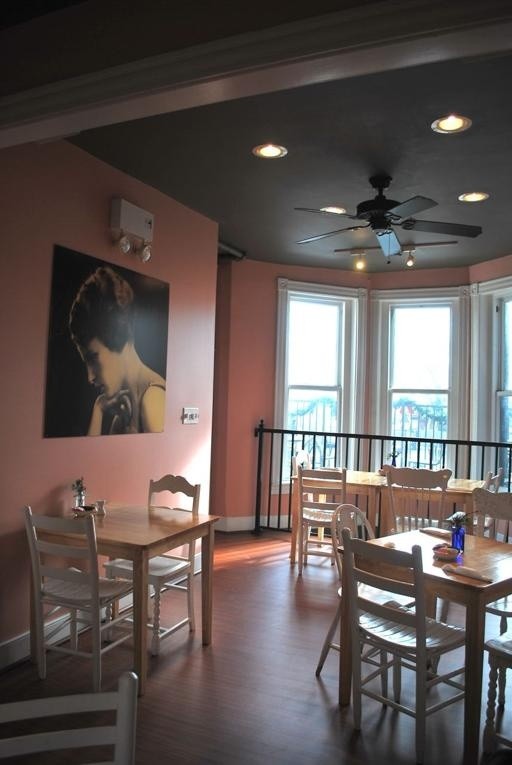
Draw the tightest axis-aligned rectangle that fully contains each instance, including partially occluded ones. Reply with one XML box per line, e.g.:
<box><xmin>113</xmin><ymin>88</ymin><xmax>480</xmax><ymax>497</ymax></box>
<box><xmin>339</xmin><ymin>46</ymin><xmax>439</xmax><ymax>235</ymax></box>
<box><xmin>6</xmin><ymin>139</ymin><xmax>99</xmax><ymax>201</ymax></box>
<box><xmin>68</xmin><ymin>266</ymin><xmax>165</xmax><ymax>436</ymax></box>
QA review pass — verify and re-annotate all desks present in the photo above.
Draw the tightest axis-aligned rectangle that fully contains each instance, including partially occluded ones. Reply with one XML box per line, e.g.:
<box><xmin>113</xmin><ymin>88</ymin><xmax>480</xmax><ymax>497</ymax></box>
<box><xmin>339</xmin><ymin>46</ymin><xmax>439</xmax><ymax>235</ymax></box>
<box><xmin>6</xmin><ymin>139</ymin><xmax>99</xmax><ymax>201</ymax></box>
<box><xmin>35</xmin><ymin>504</ymin><xmax>223</xmax><ymax>694</ymax></box>
<box><xmin>335</xmin><ymin>525</ymin><xmax>510</xmax><ymax>763</ymax></box>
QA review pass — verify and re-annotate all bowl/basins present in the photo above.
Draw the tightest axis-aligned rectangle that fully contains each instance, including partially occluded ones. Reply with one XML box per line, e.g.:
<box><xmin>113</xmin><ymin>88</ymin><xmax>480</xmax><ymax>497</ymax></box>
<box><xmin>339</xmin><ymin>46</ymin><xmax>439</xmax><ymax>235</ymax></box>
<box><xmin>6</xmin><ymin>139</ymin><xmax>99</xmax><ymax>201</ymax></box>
<box><xmin>377</xmin><ymin>470</ymin><xmax>386</xmax><ymax>476</ymax></box>
<box><xmin>432</xmin><ymin>542</ymin><xmax>460</xmax><ymax>561</ymax></box>
<box><xmin>72</xmin><ymin>506</ymin><xmax>95</xmax><ymax>519</ymax></box>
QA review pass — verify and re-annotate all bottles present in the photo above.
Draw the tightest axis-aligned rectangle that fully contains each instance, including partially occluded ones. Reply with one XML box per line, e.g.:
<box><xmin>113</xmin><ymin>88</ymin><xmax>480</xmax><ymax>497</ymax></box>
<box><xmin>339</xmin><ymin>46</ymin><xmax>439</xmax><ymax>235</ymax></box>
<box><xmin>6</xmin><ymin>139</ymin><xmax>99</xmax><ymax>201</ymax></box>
<box><xmin>95</xmin><ymin>499</ymin><xmax>107</xmax><ymax>516</ymax></box>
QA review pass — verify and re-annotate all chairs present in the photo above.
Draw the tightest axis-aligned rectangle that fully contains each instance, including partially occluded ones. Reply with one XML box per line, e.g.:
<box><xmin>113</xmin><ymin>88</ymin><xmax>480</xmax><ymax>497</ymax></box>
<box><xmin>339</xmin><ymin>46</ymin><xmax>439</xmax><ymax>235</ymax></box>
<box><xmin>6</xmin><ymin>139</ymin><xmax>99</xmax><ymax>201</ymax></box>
<box><xmin>1</xmin><ymin>672</ymin><xmax>144</xmax><ymax>765</ymax></box>
<box><xmin>340</xmin><ymin>524</ymin><xmax>464</xmax><ymax>756</ymax></box>
<box><xmin>314</xmin><ymin>502</ymin><xmax>390</xmax><ymax>695</ymax></box>
<box><xmin>480</xmin><ymin>629</ymin><xmax>512</xmax><ymax>764</ymax></box>
<box><xmin>432</xmin><ymin>487</ymin><xmax>510</xmax><ymax>678</ymax></box>
<box><xmin>99</xmin><ymin>474</ymin><xmax>200</xmax><ymax>656</ymax></box>
<box><xmin>21</xmin><ymin>505</ymin><xmax>135</xmax><ymax>691</ymax></box>
<box><xmin>287</xmin><ymin>454</ymin><xmax>505</xmax><ymax>578</ymax></box>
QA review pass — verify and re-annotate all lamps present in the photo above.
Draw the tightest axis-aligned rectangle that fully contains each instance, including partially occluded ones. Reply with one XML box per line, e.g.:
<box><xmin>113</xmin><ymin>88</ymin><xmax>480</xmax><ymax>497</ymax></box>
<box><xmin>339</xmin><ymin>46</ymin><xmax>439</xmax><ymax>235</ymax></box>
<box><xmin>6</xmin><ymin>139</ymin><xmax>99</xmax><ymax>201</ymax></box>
<box><xmin>333</xmin><ymin>239</ymin><xmax>459</xmax><ymax>270</ymax></box>
<box><xmin>108</xmin><ymin>195</ymin><xmax>157</xmax><ymax>265</ymax></box>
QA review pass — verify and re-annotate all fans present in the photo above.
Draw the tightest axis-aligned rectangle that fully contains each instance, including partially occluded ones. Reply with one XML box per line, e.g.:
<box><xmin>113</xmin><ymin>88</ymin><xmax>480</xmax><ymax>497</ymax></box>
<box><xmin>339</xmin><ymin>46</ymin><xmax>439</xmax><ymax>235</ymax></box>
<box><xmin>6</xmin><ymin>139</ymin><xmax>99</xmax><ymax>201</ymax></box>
<box><xmin>290</xmin><ymin>171</ymin><xmax>484</xmax><ymax>256</ymax></box>
